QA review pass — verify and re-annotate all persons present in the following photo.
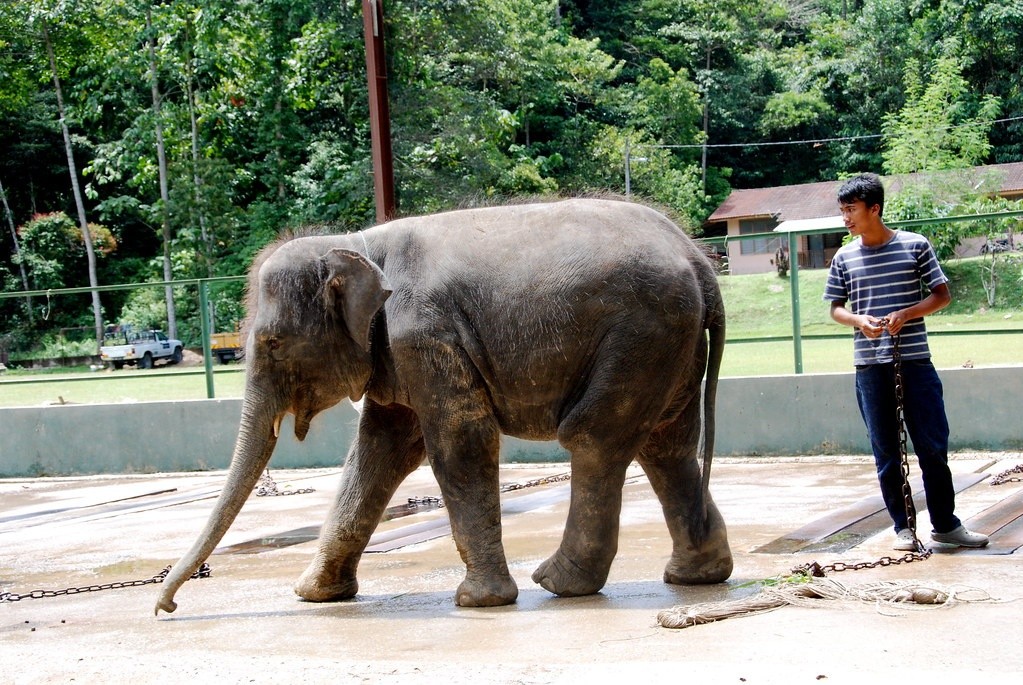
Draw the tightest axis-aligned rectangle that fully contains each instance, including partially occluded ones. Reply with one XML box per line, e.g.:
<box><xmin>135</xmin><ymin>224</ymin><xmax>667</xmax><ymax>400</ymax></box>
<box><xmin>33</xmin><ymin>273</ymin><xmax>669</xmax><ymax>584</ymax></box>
<box><xmin>824</xmin><ymin>171</ymin><xmax>990</xmax><ymax>552</ymax></box>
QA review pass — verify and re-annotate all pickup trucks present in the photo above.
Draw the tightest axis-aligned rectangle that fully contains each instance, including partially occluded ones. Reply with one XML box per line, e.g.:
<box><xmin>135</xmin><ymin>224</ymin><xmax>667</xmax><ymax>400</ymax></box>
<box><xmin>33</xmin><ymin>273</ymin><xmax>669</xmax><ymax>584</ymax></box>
<box><xmin>210</xmin><ymin>319</ymin><xmax>248</xmax><ymax>365</ymax></box>
<box><xmin>99</xmin><ymin>329</ymin><xmax>186</xmax><ymax>370</ymax></box>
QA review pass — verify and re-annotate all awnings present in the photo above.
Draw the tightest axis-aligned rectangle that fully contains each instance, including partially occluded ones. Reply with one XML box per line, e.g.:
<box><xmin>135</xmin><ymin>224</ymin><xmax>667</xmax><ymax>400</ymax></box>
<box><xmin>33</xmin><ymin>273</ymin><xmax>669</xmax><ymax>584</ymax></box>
<box><xmin>773</xmin><ymin>215</ymin><xmax>848</xmax><ymax>251</ymax></box>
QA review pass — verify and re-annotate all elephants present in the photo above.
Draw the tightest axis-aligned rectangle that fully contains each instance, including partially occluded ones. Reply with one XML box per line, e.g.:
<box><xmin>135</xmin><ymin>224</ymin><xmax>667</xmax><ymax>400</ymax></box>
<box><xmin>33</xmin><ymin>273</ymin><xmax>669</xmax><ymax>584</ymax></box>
<box><xmin>150</xmin><ymin>192</ymin><xmax>736</xmax><ymax>615</ymax></box>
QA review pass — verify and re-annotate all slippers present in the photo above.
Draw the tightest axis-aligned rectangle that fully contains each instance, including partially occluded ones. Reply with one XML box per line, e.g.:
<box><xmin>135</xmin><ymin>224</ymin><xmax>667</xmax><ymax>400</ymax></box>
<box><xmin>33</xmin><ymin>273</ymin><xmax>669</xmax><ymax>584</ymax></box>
<box><xmin>893</xmin><ymin>528</ymin><xmax>918</xmax><ymax>550</ymax></box>
<box><xmin>930</xmin><ymin>525</ymin><xmax>989</xmax><ymax>547</ymax></box>
